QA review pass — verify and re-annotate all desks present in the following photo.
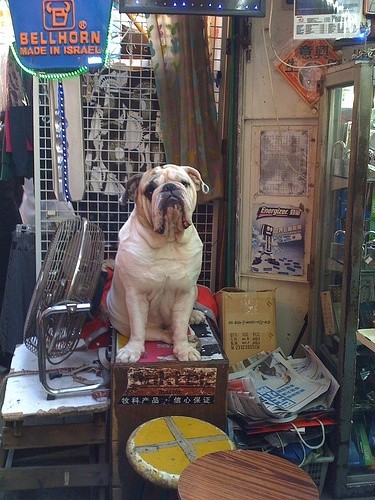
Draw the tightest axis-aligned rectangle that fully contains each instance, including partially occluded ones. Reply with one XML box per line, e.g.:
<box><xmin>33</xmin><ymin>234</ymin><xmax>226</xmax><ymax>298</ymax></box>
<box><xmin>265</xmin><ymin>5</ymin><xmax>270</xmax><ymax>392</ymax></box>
<box><xmin>109</xmin><ymin>315</ymin><xmax>230</xmax><ymax>500</ymax></box>
<box><xmin>177</xmin><ymin>448</ymin><xmax>320</xmax><ymax>500</ymax></box>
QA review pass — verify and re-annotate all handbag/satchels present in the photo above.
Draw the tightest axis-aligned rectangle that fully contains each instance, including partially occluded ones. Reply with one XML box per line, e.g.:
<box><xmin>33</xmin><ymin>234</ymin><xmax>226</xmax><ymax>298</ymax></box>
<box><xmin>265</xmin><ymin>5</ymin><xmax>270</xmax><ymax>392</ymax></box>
<box><xmin>246</xmin><ymin>411</ymin><xmax>335</xmax><ymax>467</ymax></box>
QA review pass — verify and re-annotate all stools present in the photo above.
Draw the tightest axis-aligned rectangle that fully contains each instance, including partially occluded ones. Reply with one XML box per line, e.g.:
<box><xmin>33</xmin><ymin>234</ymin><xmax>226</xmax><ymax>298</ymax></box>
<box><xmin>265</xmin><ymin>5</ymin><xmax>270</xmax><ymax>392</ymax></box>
<box><xmin>0</xmin><ymin>340</ymin><xmax>111</xmax><ymax>500</ymax></box>
<box><xmin>125</xmin><ymin>414</ymin><xmax>238</xmax><ymax>500</ymax></box>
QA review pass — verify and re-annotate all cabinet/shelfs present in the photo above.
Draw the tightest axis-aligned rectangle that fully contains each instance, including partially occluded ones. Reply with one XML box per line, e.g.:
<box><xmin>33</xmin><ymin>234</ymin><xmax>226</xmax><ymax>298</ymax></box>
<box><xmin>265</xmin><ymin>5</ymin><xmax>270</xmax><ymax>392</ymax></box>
<box><xmin>310</xmin><ymin>52</ymin><xmax>375</xmax><ymax>500</ymax></box>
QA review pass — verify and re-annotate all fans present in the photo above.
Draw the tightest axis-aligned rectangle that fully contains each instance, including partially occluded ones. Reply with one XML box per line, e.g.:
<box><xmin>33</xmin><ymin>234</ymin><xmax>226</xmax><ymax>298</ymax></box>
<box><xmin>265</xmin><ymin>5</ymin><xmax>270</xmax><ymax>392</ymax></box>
<box><xmin>22</xmin><ymin>215</ymin><xmax>106</xmax><ymax>400</ymax></box>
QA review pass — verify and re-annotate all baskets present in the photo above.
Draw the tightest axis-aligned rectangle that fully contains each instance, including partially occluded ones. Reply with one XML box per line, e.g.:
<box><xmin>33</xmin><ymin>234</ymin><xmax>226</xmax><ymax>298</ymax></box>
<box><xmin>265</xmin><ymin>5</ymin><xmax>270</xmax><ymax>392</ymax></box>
<box><xmin>300</xmin><ymin>445</ymin><xmax>334</xmax><ymax>496</ymax></box>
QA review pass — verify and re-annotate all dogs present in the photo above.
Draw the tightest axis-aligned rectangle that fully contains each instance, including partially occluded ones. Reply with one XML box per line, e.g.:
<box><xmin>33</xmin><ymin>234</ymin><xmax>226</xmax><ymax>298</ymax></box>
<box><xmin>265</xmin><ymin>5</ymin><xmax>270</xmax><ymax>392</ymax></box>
<box><xmin>105</xmin><ymin>163</ymin><xmax>209</xmax><ymax>362</ymax></box>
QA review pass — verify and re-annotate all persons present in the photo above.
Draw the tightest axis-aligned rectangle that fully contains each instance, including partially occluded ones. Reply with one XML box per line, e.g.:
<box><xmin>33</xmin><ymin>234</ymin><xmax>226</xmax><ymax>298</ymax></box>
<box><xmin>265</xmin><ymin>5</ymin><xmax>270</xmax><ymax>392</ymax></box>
<box><xmin>258</xmin><ymin>354</ymin><xmax>291</xmax><ymax>385</ymax></box>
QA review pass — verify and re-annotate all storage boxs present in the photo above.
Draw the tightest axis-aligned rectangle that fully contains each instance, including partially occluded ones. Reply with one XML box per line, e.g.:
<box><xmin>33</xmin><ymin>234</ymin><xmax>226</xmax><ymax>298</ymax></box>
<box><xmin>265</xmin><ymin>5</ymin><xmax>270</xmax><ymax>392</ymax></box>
<box><xmin>290</xmin><ymin>442</ymin><xmax>335</xmax><ymax>496</ymax></box>
<box><xmin>214</xmin><ymin>287</ymin><xmax>280</xmax><ymax>370</ymax></box>
